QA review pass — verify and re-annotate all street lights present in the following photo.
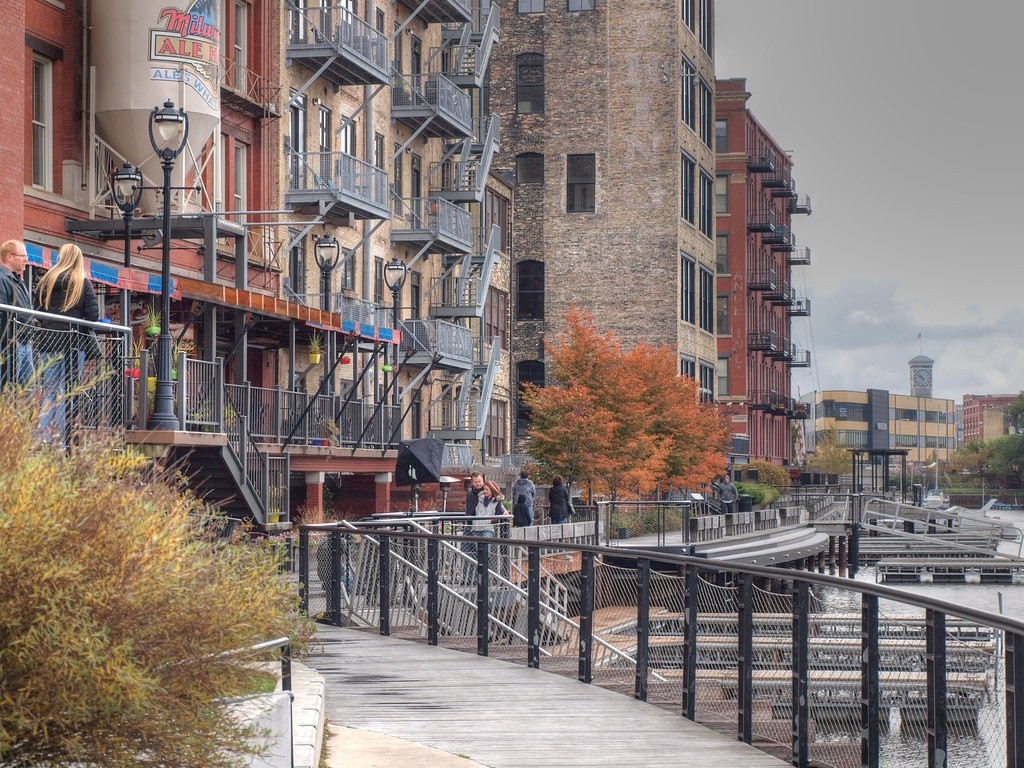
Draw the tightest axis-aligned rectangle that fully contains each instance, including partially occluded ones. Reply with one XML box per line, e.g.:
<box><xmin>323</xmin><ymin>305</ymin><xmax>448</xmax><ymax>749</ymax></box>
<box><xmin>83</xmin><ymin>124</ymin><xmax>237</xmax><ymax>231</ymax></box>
<box><xmin>109</xmin><ymin>159</ymin><xmax>143</xmax><ymax>430</ymax></box>
<box><xmin>383</xmin><ymin>257</ymin><xmax>407</xmax><ymax>443</ymax></box>
<box><xmin>313</xmin><ymin>232</ymin><xmax>341</xmax><ymax>396</ymax></box>
<box><xmin>147</xmin><ymin>98</ymin><xmax>189</xmax><ymax>430</ymax></box>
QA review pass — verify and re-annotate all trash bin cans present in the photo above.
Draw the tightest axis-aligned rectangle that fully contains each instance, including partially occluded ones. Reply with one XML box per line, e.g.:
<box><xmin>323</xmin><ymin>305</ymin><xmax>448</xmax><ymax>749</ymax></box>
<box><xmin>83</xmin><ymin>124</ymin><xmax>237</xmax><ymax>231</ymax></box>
<box><xmin>738</xmin><ymin>495</ymin><xmax>754</xmax><ymax>512</ymax></box>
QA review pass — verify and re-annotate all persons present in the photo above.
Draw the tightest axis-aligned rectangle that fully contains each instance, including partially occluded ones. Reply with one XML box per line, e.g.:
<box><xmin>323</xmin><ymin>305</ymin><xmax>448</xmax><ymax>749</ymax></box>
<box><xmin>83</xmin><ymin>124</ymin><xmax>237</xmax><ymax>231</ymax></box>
<box><xmin>468</xmin><ymin>481</ymin><xmax>504</xmax><ymax>559</ymax></box>
<box><xmin>719</xmin><ymin>475</ymin><xmax>737</xmax><ymax>513</ymax></box>
<box><xmin>461</xmin><ymin>472</ymin><xmax>508</xmax><ymax>586</ymax></box>
<box><xmin>34</xmin><ymin>243</ymin><xmax>100</xmax><ymax>449</ymax></box>
<box><xmin>548</xmin><ymin>476</ymin><xmax>569</xmax><ymax>524</ymax></box>
<box><xmin>513</xmin><ymin>495</ymin><xmax>532</xmax><ymax>527</ymax></box>
<box><xmin>0</xmin><ymin>239</ymin><xmax>35</xmax><ymax>388</ymax></box>
<box><xmin>316</xmin><ymin>531</ymin><xmax>345</xmax><ymax>611</ymax></box>
<box><xmin>515</xmin><ymin>471</ymin><xmax>536</xmax><ymax>525</ymax></box>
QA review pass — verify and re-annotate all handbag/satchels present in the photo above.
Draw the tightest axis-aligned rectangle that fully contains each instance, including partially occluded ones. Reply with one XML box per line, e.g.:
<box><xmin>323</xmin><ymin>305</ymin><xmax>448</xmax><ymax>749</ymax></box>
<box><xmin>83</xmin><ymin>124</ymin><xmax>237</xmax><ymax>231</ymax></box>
<box><xmin>567</xmin><ymin>502</ymin><xmax>576</xmax><ymax>514</ymax></box>
